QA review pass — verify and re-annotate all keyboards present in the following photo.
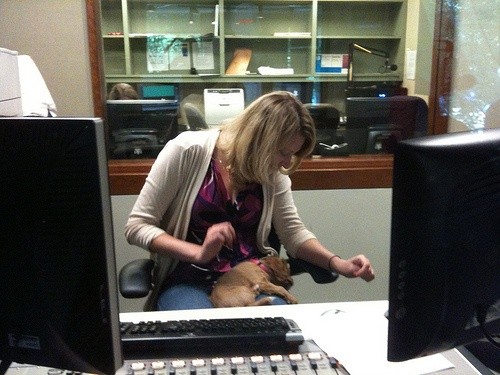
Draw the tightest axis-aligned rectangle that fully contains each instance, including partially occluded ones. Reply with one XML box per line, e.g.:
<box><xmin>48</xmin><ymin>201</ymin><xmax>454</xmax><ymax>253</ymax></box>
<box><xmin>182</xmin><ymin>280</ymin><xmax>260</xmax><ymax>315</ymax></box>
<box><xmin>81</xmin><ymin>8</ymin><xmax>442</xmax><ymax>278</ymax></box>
<box><xmin>119</xmin><ymin>317</ymin><xmax>304</xmax><ymax>349</ymax></box>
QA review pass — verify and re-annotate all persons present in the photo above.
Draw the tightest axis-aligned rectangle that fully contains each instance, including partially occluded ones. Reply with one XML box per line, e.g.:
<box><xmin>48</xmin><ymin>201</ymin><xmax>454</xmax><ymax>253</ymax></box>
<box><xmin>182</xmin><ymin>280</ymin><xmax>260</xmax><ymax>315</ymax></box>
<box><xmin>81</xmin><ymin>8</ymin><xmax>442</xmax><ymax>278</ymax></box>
<box><xmin>124</xmin><ymin>91</ymin><xmax>375</xmax><ymax>312</ymax></box>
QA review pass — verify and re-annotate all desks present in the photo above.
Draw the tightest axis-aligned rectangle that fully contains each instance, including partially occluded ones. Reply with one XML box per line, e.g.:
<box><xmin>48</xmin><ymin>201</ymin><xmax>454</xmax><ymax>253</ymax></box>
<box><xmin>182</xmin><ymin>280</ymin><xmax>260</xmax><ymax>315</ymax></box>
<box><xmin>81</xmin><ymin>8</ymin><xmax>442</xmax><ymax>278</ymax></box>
<box><xmin>4</xmin><ymin>299</ymin><xmax>485</xmax><ymax>375</ymax></box>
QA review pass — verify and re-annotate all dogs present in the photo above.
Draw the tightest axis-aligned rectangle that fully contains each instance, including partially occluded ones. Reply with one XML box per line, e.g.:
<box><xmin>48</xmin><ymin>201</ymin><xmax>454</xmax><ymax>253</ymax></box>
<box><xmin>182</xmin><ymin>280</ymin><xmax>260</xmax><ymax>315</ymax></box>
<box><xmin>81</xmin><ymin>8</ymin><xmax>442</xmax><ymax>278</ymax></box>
<box><xmin>210</xmin><ymin>255</ymin><xmax>299</xmax><ymax>308</ymax></box>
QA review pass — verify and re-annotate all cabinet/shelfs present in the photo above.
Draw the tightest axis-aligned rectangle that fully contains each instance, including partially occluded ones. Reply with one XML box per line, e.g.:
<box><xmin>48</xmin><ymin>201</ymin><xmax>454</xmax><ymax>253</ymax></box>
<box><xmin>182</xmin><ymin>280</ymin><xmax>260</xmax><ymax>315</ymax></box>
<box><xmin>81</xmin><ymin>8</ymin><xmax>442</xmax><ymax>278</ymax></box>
<box><xmin>99</xmin><ymin>0</ymin><xmax>421</xmax><ymax>103</ymax></box>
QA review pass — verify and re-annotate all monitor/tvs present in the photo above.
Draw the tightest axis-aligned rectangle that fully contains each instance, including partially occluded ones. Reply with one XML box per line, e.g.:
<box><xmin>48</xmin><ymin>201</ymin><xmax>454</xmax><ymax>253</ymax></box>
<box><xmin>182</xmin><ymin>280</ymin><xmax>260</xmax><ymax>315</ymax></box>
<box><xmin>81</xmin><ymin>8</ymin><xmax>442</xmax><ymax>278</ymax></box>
<box><xmin>387</xmin><ymin>125</ymin><xmax>500</xmax><ymax>375</ymax></box>
<box><xmin>0</xmin><ymin>116</ymin><xmax>123</xmax><ymax>375</ymax></box>
<box><xmin>106</xmin><ymin>99</ymin><xmax>181</xmax><ymax>160</ymax></box>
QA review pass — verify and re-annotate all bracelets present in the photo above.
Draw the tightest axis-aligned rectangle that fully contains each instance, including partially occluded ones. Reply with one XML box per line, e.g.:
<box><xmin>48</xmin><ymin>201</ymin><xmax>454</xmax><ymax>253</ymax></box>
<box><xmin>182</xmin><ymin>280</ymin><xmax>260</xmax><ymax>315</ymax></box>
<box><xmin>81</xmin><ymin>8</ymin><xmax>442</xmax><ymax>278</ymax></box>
<box><xmin>328</xmin><ymin>255</ymin><xmax>341</xmax><ymax>272</ymax></box>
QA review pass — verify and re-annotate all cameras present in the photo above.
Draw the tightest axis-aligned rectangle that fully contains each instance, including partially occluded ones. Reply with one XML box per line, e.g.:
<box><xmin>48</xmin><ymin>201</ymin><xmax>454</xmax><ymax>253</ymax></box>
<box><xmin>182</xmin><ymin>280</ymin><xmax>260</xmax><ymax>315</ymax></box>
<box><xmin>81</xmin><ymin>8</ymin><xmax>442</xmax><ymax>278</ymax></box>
<box><xmin>347</xmin><ymin>97</ymin><xmax>430</xmax><ymax>155</ymax></box>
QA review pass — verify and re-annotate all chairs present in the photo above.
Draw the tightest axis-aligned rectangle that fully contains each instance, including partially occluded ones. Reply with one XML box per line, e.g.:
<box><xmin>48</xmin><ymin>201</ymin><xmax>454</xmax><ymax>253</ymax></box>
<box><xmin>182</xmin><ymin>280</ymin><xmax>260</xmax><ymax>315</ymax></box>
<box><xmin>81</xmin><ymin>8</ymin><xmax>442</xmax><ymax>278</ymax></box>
<box><xmin>119</xmin><ymin>225</ymin><xmax>339</xmax><ymax>300</ymax></box>
